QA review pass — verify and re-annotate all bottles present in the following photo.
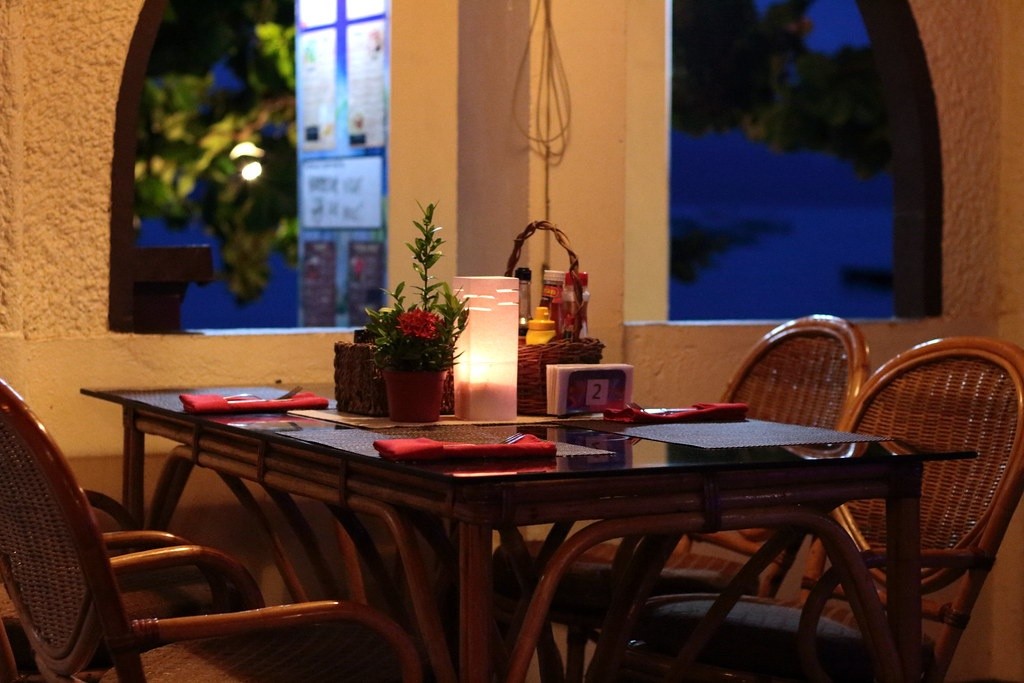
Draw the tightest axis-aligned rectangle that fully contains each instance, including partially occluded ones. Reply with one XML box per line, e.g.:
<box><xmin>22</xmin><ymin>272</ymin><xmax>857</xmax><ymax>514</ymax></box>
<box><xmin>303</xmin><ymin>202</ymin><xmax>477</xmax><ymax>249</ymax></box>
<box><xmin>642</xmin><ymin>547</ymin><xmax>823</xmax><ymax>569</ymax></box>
<box><xmin>539</xmin><ymin>269</ymin><xmax>564</xmax><ymax>342</ymax></box>
<box><xmin>525</xmin><ymin>307</ymin><xmax>555</xmax><ymax>344</ymax></box>
<box><xmin>561</xmin><ymin>272</ymin><xmax>590</xmax><ymax>340</ymax></box>
<box><xmin>514</xmin><ymin>267</ymin><xmax>531</xmax><ymax>344</ymax></box>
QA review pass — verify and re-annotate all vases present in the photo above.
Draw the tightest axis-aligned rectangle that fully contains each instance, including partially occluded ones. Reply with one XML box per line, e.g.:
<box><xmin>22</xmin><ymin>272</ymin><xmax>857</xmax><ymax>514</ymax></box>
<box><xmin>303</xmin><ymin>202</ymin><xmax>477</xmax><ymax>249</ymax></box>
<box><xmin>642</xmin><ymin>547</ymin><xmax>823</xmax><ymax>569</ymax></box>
<box><xmin>381</xmin><ymin>371</ymin><xmax>446</xmax><ymax>423</ymax></box>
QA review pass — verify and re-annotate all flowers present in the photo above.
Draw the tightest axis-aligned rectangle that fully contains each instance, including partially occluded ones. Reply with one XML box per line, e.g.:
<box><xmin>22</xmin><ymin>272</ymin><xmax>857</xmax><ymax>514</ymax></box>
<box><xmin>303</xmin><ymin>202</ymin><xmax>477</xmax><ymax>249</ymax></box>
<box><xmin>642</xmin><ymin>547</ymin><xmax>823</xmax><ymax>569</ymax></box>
<box><xmin>371</xmin><ymin>202</ymin><xmax>471</xmax><ymax>367</ymax></box>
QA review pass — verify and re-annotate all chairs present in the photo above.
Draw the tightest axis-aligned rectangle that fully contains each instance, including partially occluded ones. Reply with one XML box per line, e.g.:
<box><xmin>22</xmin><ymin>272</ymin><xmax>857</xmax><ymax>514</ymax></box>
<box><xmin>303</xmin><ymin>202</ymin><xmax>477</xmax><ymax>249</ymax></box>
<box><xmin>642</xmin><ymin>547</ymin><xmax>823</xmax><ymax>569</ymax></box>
<box><xmin>492</xmin><ymin>314</ymin><xmax>870</xmax><ymax>683</ymax></box>
<box><xmin>0</xmin><ymin>380</ymin><xmax>418</xmax><ymax>682</ymax></box>
<box><xmin>609</xmin><ymin>335</ymin><xmax>1024</xmax><ymax>683</ymax></box>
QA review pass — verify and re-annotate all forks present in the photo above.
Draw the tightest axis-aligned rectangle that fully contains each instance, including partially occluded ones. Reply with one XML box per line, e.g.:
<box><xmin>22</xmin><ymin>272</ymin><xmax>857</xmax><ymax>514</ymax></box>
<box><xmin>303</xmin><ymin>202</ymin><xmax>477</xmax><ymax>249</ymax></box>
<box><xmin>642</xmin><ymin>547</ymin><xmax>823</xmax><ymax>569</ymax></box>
<box><xmin>498</xmin><ymin>432</ymin><xmax>525</xmax><ymax>443</ymax></box>
<box><xmin>223</xmin><ymin>385</ymin><xmax>303</xmax><ymax>400</ymax></box>
<box><xmin>625</xmin><ymin>402</ymin><xmax>648</xmax><ymax>413</ymax></box>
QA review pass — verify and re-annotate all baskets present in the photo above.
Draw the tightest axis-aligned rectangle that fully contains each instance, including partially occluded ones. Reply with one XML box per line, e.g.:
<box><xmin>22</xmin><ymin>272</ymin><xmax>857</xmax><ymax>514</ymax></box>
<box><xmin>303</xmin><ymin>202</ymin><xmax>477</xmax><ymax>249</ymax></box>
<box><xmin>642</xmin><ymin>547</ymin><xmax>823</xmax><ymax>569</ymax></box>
<box><xmin>333</xmin><ymin>338</ymin><xmax>456</xmax><ymax>415</ymax></box>
<box><xmin>505</xmin><ymin>220</ymin><xmax>604</xmax><ymax>414</ymax></box>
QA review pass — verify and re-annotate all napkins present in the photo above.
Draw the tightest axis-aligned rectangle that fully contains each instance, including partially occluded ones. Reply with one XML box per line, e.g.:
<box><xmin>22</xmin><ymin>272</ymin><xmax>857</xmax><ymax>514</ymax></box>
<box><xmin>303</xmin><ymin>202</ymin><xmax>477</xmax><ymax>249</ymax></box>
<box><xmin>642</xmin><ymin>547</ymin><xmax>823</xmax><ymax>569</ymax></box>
<box><xmin>596</xmin><ymin>401</ymin><xmax>750</xmax><ymax>422</ymax></box>
<box><xmin>181</xmin><ymin>392</ymin><xmax>329</xmax><ymax>411</ymax></box>
<box><xmin>371</xmin><ymin>435</ymin><xmax>560</xmax><ymax>461</ymax></box>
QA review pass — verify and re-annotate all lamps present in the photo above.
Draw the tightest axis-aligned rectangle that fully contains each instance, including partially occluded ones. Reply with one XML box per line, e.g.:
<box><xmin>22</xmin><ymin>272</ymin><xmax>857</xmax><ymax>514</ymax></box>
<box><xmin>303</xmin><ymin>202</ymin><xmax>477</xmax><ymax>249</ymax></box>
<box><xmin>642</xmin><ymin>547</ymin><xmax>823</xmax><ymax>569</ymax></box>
<box><xmin>451</xmin><ymin>274</ymin><xmax>519</xmax><ymax>421</ymax></box>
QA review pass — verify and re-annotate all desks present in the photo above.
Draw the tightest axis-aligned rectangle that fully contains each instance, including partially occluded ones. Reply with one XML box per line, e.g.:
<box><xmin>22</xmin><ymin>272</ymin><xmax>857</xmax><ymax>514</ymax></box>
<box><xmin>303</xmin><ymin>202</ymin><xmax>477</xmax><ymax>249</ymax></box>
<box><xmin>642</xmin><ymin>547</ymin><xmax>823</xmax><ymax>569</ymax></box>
<box><xmin>78</xmin><ymin>389</ymin><xmax>926</xmax><ymax>682</ymax></box>
